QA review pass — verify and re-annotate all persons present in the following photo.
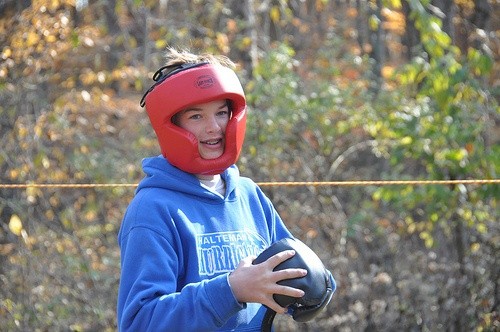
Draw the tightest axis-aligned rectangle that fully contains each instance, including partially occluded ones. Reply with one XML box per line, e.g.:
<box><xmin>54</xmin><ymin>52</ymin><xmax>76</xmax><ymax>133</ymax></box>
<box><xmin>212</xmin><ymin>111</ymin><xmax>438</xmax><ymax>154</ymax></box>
<box><xmin>113</xmin><ymin>46</ymin><xmax>337</xmax><ymax>330</ymax></box>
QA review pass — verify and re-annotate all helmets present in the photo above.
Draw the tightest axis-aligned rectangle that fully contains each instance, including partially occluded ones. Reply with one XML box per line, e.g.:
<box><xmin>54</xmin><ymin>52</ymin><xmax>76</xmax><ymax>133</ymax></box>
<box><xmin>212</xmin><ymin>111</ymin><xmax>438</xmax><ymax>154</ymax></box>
<box><xmin>138</xmin><ymin>59</ymin><xmax>248</xmax><ymax>176</ymax></box>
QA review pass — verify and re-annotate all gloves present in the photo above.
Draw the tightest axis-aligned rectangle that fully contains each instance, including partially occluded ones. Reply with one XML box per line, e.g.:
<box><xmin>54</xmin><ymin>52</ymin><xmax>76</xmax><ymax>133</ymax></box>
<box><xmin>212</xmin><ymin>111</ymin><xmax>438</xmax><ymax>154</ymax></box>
<box><xmin>251</xmin><ymin>237</ymin><xmax>333</xmax><ymax>322</ymax></box>
